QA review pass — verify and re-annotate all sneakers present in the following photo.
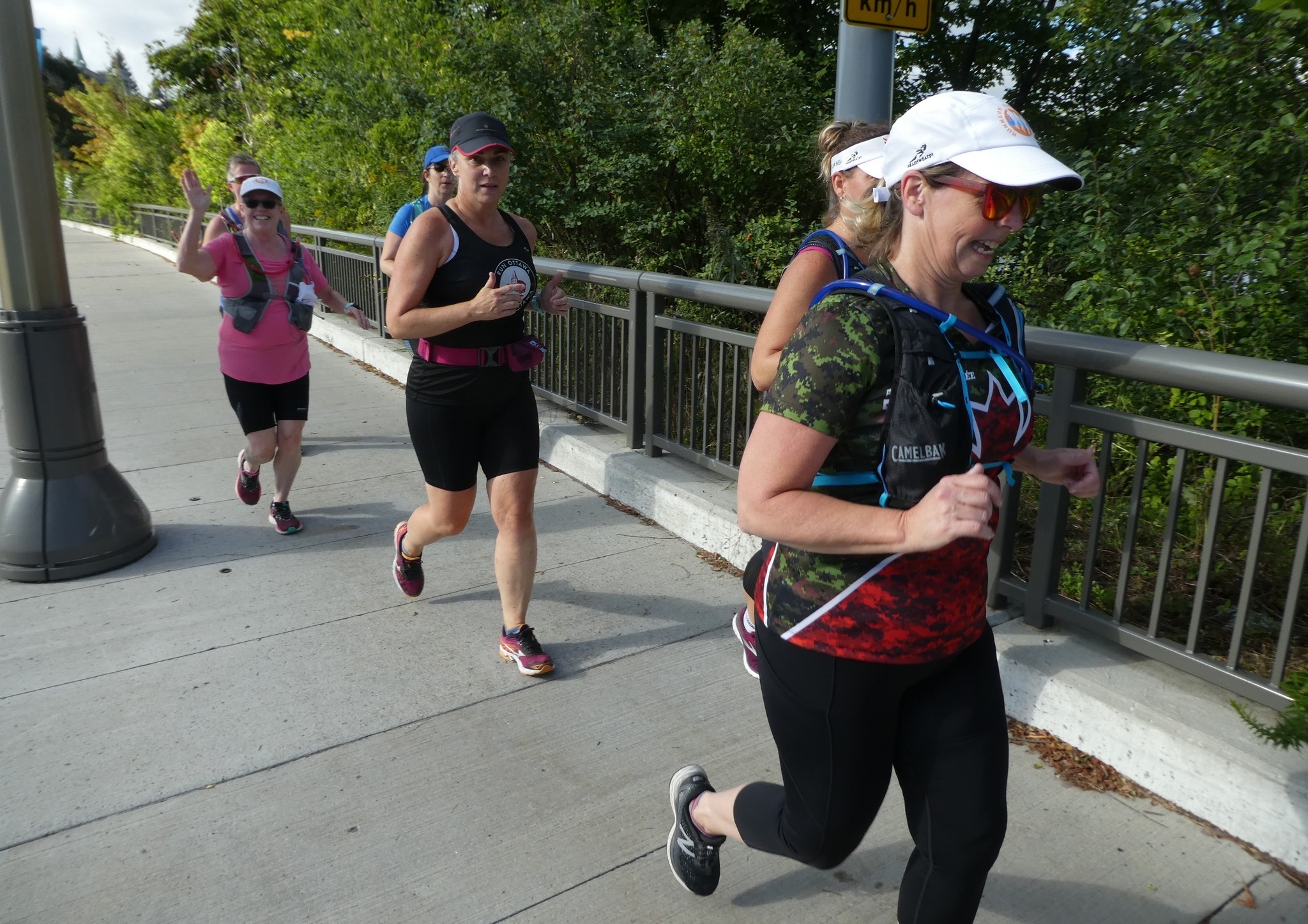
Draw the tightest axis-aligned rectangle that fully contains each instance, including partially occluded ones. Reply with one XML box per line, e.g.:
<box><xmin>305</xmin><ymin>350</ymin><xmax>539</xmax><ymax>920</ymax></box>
<box><xmin>731</xmin><ymin>607</ymin><xmax>759</xmax><ymax>679</ymax></box>
<box><xmin>392</xmin><ymin>520</ymin><xmax>425</xmax><ymax>597</ymax></box>
<box><xmin>665</xmin><ymin>764</ymin><xmax>727</xmax><ymax>898</ymax></box>
<box><xmin>268</xmin><ymin>502</ymin><xmax>304</xmax><ymax>534</ymax></box>
<box><xmin>498</xmin><ymin>624</ymin><xmax>556</xmax><ymax>675</ymax></box>
<box><xmin>236</xmin><ymin>449</ymin><xmax>261</xmax><ymax>506</ymax></box>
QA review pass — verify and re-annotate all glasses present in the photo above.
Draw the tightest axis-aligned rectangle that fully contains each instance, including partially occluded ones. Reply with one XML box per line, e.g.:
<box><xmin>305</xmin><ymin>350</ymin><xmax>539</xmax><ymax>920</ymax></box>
<box><xmin>933</xmin><ymin>173</ymin><xmax>1040</xmax><ymax>223</ymax></box>
<box><xmin>242</xmin><ymin>198</ymin><xmax>283</xmax><ymax>210</ymax></box>
<box><xmin>228</xmin><ymin>174</ymin><xmax>262</xmax><ymax>185</ymax></box>
<box><xmin>426</xmin><ymin>163</ymin><xmax>450</xmax><ymax>172</ymax></box>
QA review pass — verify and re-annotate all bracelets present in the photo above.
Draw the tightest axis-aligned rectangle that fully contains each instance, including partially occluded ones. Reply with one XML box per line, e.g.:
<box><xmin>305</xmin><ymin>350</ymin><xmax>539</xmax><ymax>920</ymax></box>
<box><xmin>532</xmin><ymin>291</ymin><xmax>546</xmax><ymax>317</ymax></box>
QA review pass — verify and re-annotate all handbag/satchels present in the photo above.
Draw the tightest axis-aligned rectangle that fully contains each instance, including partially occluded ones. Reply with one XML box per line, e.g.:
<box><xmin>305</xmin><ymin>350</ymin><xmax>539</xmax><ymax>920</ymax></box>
<box><xmin>417</xmin><ymin>334</ymin><xmax>546</xmax><ymax>372</ymax></box>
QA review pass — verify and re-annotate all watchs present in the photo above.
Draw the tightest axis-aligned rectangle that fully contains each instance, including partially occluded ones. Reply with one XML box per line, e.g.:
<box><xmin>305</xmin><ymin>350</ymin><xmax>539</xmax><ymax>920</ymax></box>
<box><xmin>344</xmin><ymin>303</ymin><xmax>359</xmax><ymax>315</ymax></box>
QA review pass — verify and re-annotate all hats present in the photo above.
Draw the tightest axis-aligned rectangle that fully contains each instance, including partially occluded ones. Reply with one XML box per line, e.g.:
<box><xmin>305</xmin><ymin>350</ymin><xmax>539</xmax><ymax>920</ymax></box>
<box><xmin>450</xmin><ymin>110</ymin><xmax>518</xmax><ymax>157</ymax></box>
<box><xmin>424</xmin><ymin>146</ymin><xmax>450</xmax><ymax>169</ymax></box>
<box><xmin>240</xmin><ymin>176</ymin><xmax>283</xmax><ymax>199</ymax></box>
<box><xmin>882</xmin><ymin>90</ymin><xmax>1085</xmax><ymax>192</ymax></box>
<box><xmin>832</xmin><ymin>134</ymin><xmax>887</xmax><ymax>180</ymax></box>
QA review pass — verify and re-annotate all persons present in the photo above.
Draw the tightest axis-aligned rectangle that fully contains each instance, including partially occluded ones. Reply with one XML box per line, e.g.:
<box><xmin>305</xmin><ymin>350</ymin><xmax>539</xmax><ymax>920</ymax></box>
<box><xmin>387</xmin><ymin>112</ymin><xmax>569</xmax><ymax>678</ymax></box>
<box><xmin>730</xmin><ymin>116</ymin><xmax>889</xmax><ymax>684</ymax></box>
<box><xmin>380</xmin><ymin>145</ymin><xmax>455</xmax><ymax>357</ymax></box>
<box><xmin>665</xmin><ymin>90</ymin><xmax>1101</xmax><ymax>924</ymax></box>
<box><xmin>174</xmin><ymin>165</ymin><xmax>369</xmax><ymax>536</ymax></box>
<box><xmin>170</xmin><ymin>155</ymin><xmax>305</xmax><ymax>457</ymax></box>
